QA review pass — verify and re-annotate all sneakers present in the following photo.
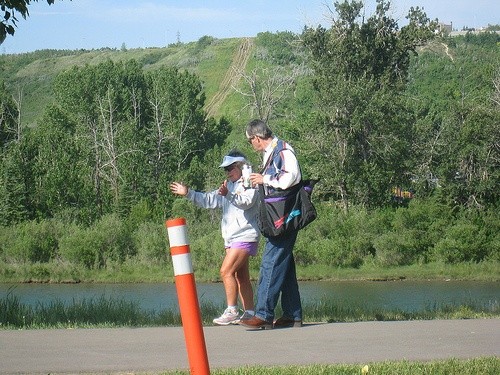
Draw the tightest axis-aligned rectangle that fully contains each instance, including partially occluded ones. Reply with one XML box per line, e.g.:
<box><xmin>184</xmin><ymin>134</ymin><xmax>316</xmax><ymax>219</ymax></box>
<box><xmin>236</xmin><ymin>310</ymin><xmax>257</xmax><ymax>325</ymax></box>
<box><xmin>212</xmin><ymin>308</ymin><xmax>240</xmax><ymax>326</ymax></box>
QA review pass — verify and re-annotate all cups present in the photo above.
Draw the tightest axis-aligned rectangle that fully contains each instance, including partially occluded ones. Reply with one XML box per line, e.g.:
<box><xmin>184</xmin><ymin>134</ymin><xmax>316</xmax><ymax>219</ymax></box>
<box><xmin>242</xmin><ymin>164</ymin><xmax>252</xmax><ymax>187</ymax></box>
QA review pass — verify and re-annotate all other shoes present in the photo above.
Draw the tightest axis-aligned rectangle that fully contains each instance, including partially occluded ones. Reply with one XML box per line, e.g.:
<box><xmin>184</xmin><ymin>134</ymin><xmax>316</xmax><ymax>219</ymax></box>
<box><xmin>238</xmin><ymin>316</ymin><xmax>273</xmax><ymax>330</ymax></box>
<box><xmin>272</xmin><ymin>319</ymin><xmax>303</xmax><ymax>328</ymax></box>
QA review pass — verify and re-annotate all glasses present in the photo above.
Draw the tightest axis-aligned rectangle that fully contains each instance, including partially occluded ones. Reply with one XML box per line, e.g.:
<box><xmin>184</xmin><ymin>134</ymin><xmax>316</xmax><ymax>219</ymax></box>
<box><xmin>248</xmin><ymin>136</ymin><xmax>254</xmax><ymax>145</ymax></box>
<box><xmin>223</xmin><ymin>164</ymin><xmax>238</xmax><ymax>171</ymax></box>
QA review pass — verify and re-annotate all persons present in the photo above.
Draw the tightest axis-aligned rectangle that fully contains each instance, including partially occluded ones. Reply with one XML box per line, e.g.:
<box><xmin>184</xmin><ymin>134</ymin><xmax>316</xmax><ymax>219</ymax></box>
<box><xmin>238</xmin><ymin>119</ymin><xmax>303</xmax><ymax>330</ymax></box>
<box><xmin>170</xmin><ymin>149</ymin><xmax>262</xmax><ymax>325</ymax></box>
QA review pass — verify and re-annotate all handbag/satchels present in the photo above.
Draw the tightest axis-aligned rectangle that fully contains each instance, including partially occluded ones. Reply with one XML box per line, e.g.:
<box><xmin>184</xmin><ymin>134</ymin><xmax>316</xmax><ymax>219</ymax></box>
<box><xmin>255</xmin><ymin>150</ymin><xmax>320</xmax><ymax>239</ymax></box>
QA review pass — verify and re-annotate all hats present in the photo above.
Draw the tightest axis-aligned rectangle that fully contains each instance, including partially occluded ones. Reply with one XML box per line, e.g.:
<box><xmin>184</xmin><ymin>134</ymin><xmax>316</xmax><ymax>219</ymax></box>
<box><xmin>217</xmin><ymin>155</ymin><xmax>247</xmax><ymax>170</ymax></box>
